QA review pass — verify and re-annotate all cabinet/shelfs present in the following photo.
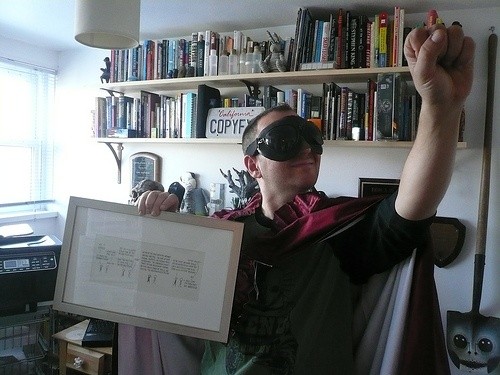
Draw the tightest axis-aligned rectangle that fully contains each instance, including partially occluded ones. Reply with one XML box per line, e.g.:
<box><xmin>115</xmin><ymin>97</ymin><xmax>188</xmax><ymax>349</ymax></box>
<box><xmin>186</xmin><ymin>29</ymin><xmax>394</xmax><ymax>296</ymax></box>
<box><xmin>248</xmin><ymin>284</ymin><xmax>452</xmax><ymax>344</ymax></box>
<box><xmin>98</xmin><ymin>66</ymin><xmax>465</xmax><ymax>183</ymax></box>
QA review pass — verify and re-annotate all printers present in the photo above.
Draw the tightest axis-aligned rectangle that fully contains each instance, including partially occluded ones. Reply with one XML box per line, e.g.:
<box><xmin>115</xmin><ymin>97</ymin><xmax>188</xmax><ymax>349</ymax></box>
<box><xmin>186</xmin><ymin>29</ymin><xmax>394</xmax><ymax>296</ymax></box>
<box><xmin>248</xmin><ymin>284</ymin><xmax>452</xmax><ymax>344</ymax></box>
<box><xmin>0</xmin><ymin>234</ymin><xmax>62</xmax><ymax>301</ymax></box>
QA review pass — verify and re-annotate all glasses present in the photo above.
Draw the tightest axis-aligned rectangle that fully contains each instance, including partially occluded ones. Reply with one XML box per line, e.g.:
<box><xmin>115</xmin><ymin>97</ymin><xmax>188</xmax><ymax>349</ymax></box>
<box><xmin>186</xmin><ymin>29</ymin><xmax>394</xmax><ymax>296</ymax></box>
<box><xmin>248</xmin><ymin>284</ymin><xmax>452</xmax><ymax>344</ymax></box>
<box><xmin>245</xmin><ymin>115</ymin><xmax>324</xmax><ymax>161</ymax></box>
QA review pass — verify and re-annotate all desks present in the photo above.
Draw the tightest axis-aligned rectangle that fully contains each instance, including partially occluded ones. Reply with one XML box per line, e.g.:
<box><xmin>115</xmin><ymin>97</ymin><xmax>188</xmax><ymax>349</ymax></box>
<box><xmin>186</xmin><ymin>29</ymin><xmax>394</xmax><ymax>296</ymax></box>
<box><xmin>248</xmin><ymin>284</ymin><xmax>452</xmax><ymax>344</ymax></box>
<box><xmin>52</xmin><ymin>318</ymin><xmax>113</xmax><ymax>375</ymax></box>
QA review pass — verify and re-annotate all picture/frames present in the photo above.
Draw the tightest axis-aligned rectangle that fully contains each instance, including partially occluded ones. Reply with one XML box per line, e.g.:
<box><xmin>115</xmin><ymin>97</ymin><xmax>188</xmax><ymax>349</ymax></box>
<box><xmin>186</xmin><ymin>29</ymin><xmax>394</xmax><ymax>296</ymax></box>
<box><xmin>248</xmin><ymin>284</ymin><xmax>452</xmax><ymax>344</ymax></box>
<box><xmin>357</xmin><ymin>178</ymin><xmax>400</xmax><ymax>198</ymax></box>
<box><xmin>52</xmin><ymin>196</ymin><xmax>245</xmax><ymax>343</ymax></box>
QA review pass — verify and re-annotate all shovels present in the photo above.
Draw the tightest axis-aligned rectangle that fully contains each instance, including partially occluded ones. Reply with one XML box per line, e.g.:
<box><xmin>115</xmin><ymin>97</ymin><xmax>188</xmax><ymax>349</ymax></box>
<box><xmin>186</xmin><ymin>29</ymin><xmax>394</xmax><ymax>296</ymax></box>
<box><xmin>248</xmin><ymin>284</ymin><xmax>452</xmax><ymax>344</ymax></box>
<box><xmin>445</xmin><ymin>32</ymin><xmax>500</xmax><ymax>375</ymax></box>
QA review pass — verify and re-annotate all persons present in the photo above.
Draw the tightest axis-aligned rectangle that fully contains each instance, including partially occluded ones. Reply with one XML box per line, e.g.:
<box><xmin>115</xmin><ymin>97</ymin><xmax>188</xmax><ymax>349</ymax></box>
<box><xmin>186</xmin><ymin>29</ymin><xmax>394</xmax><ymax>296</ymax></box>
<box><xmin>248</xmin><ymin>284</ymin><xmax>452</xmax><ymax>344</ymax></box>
<box><xmin>134</xmin><ymin>23</ymin><xmax>476</xmax><ymax>375</ymax></box>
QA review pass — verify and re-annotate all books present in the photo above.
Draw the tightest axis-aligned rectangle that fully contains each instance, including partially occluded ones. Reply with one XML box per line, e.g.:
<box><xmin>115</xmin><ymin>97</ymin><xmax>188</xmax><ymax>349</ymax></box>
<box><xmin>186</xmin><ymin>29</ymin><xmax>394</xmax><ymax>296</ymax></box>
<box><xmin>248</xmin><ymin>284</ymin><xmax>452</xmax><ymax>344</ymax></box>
<box><xmin>95</xmin><ymin>4</ymin><xmax>464</xmax><ymax>137</ymax></box>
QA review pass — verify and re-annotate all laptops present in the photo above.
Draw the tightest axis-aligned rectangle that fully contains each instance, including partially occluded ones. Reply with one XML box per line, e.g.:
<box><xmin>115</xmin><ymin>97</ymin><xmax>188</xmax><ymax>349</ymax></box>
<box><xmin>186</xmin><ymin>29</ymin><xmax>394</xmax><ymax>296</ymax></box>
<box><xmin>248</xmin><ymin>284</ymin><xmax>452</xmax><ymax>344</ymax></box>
<box><xmin>82</xmin><ymin>318</ymin><xmax>114</xmax><ymax>347</ymax></box>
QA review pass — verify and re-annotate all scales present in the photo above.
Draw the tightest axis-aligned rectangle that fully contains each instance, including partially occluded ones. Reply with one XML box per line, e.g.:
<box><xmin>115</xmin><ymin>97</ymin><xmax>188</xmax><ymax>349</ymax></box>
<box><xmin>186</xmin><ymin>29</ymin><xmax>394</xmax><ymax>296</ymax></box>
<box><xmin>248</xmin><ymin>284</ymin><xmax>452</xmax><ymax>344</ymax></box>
<box><xmin>0</xmin><ymin>234</ymin><xmax>62</xmax><ymax>275</ymax></box>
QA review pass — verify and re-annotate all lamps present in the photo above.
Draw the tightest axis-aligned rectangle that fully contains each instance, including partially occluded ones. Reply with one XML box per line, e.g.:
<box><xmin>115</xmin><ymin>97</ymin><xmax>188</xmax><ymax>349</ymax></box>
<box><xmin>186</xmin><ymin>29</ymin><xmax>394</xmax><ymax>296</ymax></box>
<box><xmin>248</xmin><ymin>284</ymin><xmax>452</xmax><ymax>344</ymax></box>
<box><xmin>76</xmin><ymin>0</ymin><xmax>139</xmax><ymax>50</ymax></box>
